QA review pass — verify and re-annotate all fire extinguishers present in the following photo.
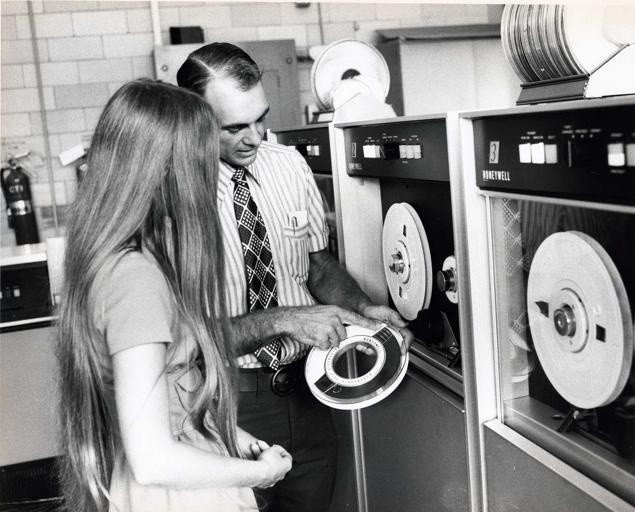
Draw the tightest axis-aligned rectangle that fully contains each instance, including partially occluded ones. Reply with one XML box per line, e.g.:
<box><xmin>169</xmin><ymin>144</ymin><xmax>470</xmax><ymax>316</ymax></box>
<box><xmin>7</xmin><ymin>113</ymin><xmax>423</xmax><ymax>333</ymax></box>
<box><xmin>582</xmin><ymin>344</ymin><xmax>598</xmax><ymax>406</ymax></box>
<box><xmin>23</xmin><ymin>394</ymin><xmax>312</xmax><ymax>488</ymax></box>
<box><xmin>1</xmin><ymin>152</ymin><xmax>40</xmax><ymax>245</ymax></box>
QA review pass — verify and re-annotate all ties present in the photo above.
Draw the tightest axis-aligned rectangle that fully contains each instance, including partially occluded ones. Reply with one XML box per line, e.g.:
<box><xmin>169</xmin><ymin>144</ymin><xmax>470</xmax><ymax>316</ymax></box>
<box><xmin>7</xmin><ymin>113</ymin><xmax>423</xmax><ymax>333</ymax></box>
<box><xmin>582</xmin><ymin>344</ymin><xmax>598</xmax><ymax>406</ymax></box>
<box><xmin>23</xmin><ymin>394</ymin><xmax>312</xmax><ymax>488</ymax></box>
<box><xmin>231</xmin><ymin>170</ymin><xmax>280</xmax><ymax>370</ymax></box>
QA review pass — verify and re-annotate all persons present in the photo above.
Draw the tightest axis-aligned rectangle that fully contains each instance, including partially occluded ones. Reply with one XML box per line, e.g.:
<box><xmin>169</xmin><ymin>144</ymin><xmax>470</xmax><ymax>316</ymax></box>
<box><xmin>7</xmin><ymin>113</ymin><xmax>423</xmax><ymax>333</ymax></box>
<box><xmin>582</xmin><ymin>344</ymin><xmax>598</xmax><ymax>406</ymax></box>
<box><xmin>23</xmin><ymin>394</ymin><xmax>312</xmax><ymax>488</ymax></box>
<box><xmin>176</xmin><ymin>42</ymin><xmax>414</xmax><ymax>510</ymax></box>
<box><xmin>53</xmin><ymin>77</ymin><xmax>293</xmax><ymax>511</ymax></box>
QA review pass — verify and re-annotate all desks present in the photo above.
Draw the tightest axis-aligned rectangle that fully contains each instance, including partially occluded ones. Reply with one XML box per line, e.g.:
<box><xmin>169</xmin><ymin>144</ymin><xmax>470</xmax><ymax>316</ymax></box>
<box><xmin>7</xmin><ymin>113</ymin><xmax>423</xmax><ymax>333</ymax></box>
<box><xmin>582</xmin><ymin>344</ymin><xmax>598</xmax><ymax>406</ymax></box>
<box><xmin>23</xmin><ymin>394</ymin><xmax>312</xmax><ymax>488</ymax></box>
<box><xmin>0</xmin><ymin>236</ymin><xmax>69</xmax><ymax>508</ymax></box>
